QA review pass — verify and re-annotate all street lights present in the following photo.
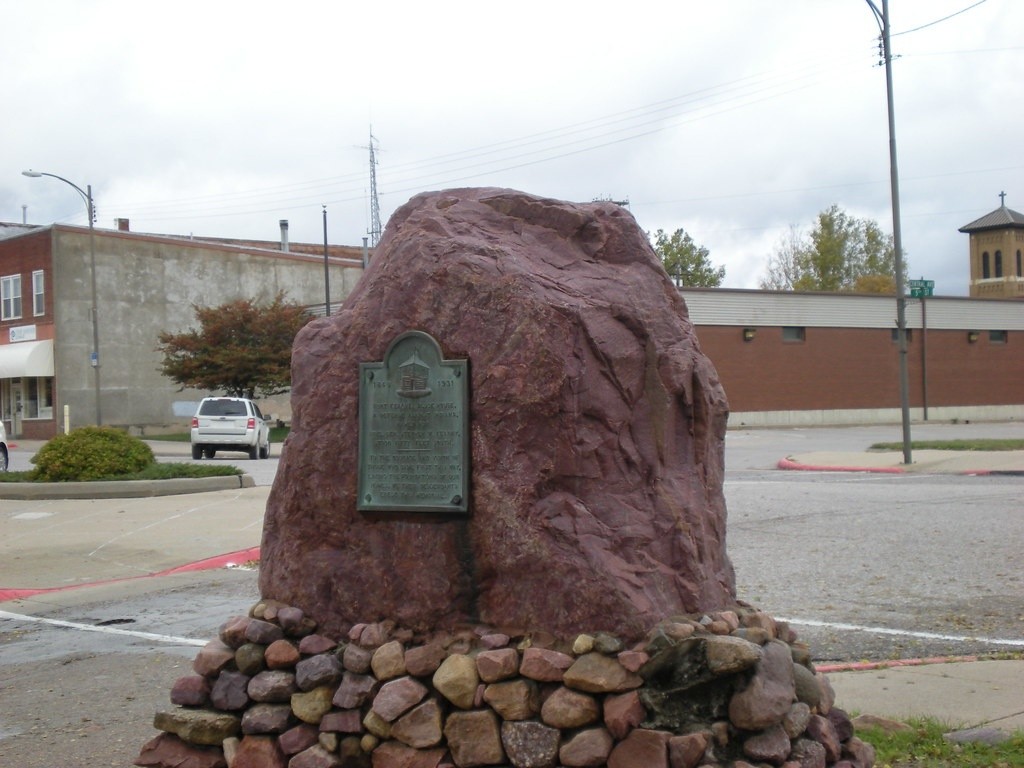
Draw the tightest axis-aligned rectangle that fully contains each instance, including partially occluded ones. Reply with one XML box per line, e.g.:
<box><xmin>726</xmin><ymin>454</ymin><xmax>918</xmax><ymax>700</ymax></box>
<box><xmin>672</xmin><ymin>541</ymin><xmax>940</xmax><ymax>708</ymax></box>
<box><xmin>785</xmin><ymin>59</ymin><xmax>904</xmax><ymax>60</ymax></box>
<box><xmin>20</xmin><ymin>170</ymin><xmax>101</xmax><ymax>434</ymax></box>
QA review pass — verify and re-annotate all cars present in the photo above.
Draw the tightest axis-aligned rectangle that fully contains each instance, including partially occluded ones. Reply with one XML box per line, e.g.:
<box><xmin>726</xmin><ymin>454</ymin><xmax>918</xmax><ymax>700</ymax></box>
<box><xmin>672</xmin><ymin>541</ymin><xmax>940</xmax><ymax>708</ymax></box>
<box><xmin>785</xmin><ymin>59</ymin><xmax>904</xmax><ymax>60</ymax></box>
<box><xmin>0</xmin><ymin>421</ymin><xmax>10</xmax><ymax>472</ymax></box>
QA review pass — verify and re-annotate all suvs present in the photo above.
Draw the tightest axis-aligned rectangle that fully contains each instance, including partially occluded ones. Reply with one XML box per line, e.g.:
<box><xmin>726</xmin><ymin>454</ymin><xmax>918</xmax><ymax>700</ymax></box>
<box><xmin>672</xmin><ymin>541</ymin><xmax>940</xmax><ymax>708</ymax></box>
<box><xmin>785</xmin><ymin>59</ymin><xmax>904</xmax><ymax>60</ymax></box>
<box><xmin>189</xmin><ymin>395</ymin><xmax>271</xmax><ymax>459</ymax></box>
